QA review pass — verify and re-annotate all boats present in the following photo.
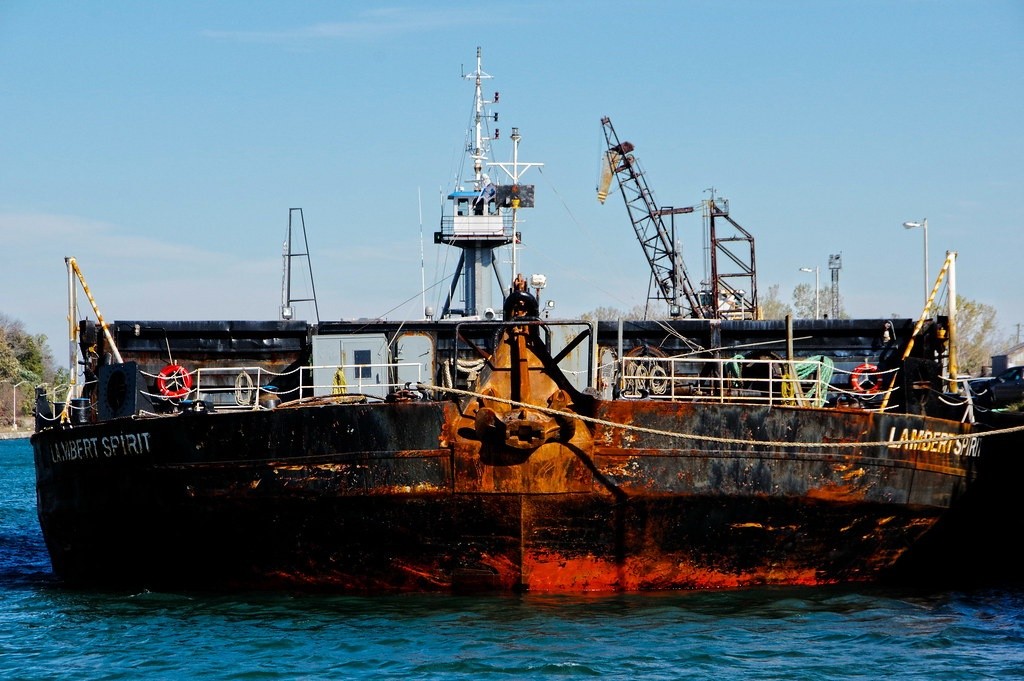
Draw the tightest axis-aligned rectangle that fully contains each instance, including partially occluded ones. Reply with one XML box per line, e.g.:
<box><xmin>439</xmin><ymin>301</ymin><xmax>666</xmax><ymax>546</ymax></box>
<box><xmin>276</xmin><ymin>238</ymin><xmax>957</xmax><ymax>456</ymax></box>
<box><xmin>30</xmin><ymin>250</ymin><xmax>1024</xmax><ymax>609</ymax></box>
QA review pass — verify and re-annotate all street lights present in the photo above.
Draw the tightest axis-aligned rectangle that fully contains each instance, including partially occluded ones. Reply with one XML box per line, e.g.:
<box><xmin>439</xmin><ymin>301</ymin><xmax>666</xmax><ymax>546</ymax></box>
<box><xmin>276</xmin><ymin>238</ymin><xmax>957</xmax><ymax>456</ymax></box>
<box><xmin>799</xmin><ymin>266</ymin><xmax>819</xmax><ymax>319</ymax></box>
<box><xmin>53</xmin><ymin>384</ymin><xmax>65</xmax><ymax>418</ymax></box>
<box><xmin>34</xmin><ymin>382</ymin><xmax>50</xmax><ymax>388</ymax></box>
<box><xmin>903</xmin><ymin>217</ymin><xmax>930</xmax><ymax>320</ymax></box>
<box><xmin>13</xmin><ymin>381</ymin><xmax>28</xmax><ymax>430</ymax></box>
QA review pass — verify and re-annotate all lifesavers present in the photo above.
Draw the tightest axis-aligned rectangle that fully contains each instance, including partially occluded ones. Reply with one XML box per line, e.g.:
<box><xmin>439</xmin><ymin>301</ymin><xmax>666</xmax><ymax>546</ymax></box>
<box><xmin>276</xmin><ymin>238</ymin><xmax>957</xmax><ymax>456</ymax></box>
<box><xmin>158</xmin><ymin>363</ymin><xmax>192</xmax><ymax>400</ymax></box>
<box><xmin>852</xmin><ymin>364</ymin><xmax>882</xmax><ymax>396</ymax></box>
